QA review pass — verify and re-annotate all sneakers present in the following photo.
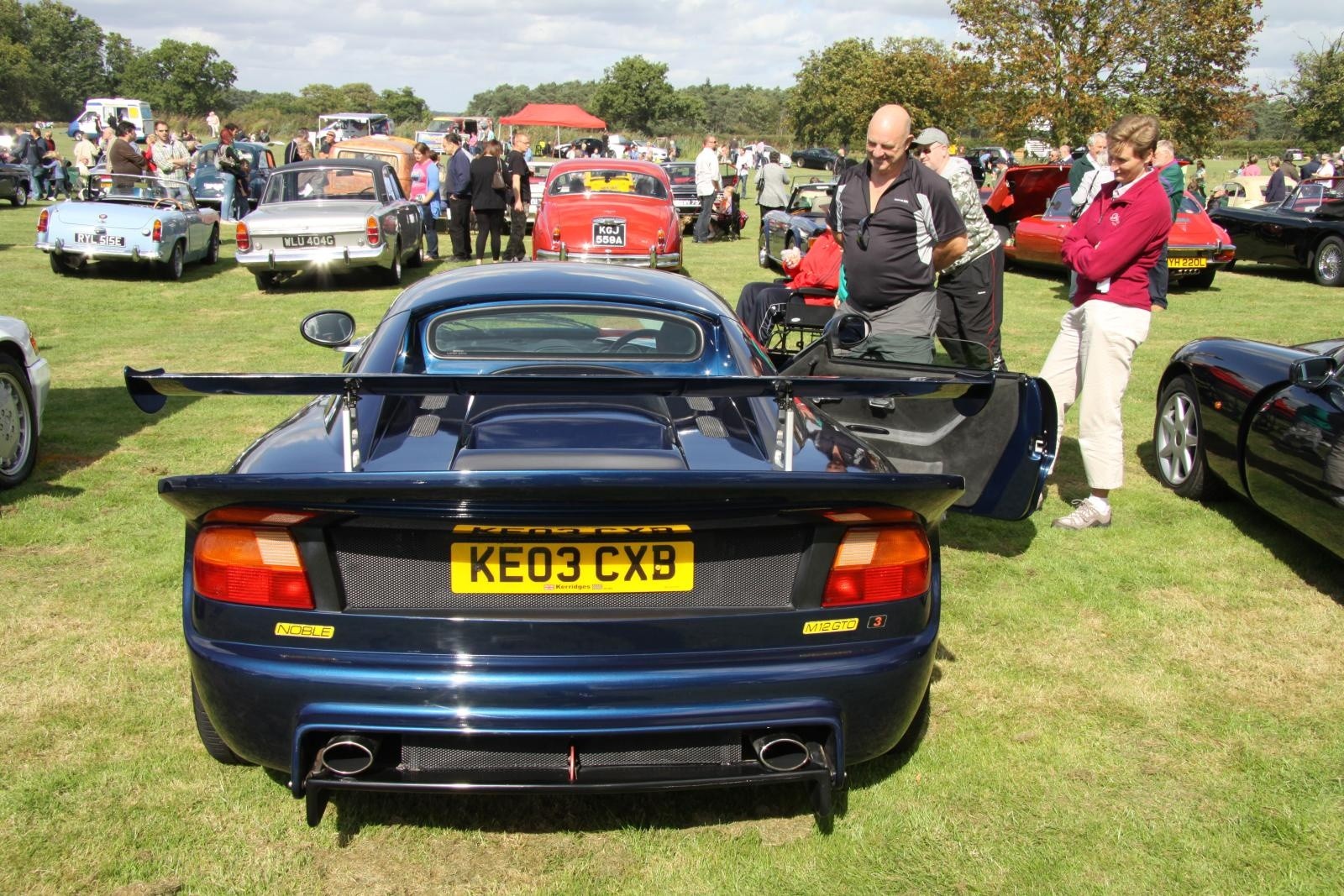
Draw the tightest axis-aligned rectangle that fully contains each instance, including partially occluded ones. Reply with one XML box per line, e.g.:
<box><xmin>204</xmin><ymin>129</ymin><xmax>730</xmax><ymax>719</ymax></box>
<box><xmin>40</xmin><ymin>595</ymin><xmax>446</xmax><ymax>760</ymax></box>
<box><xmin>1051</xmin><ymin>497</ymin><xmax>1112</xmax><ymax>530</ymax></box>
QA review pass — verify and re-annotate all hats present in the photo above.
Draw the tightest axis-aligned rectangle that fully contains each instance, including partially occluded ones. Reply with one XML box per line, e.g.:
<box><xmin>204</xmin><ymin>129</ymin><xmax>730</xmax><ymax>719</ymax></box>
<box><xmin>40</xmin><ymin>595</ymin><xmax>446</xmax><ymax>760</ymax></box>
<box><xmin>909</xmin><ymin>127</ymin><xmax>950</xmax><ymax>150</ymax></box>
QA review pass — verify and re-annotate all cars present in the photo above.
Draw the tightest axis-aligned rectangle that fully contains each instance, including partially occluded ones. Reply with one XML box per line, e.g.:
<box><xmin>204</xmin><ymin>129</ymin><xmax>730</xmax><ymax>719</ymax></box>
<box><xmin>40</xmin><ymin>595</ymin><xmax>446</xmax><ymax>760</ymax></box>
<box><xmin>0</xmin><ymin>316</ymin><xmax>52</xmax><ymax>490</ymax></box>
<box><xmin>0</xmin><ymin>124</ymin><xmax>32</xmax><ymax>209</ymax></box>
<box><xmin>791</xmin><ymin>147</ymin><xmax>838</xmax><ymax>171</ymax></box>
<box><xmin>234</xmin><ymin>157</ymin><xmax>426</xmax><ymax>291</ymax></box>
<box><xmin>88</xmin><ymin>152</ymin><xmax>112</xmax><ymax>192</ymax></box>
<box><xmin>188</xmin><ymin>140</ymin><xmax>281</xmax><ymax>210</ymax></box>
<box><xmin>743</xmin><ymin>144</ymin><xmax>793</xmax><ymax>169</ymax></box>
<box><xmin>531</xmin><ymin>157</ymin><xmax>684</xmax><ymax>276</ymax></box>
<box><xmin>499</xmin><ymin>162</ymin><xmax>575</xmax><ymax>235</ymax></box>
<box><xmin>650</xmin><ymin>161</ymin><xmax>741</xmax><ymax>218</ymax></box>
<box><xmin>962</xmin><ymin>146</ymin><xmax>1019</xmax><ymax>181</ymax></box>
<box><xmin>607</xmin><ymin>135</ymin><xmax>671</xmax><ymax>163</ymax></box>
<box><xmin>552</xmin><ymin>138</ymin><xmax>603</xmax><ymax>160</ymax></box>
<box><xmin>317</xmin><ymin>134</ymin><xmax>418</xmax><ymax>201</ymax></box>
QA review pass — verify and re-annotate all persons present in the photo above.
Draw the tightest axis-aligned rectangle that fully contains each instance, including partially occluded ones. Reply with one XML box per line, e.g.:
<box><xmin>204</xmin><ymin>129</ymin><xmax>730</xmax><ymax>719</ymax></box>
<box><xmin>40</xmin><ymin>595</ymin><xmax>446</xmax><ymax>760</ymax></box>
<box><xmin>910</xmin><ymin>128</ymin><xmax>1007</xmax><ymax>371</ymax></box>
<box><xmin>0</xmin><ymin>121</ymin><xmax>72</xmax><ymax>202</ymax></box>
<box><xmin>1265</xmin><ymin>145</ymin><xmax>1344</xmax><ymax>203</ymax></box>
<box><xmin>822</xmin><ymin>103</ymin><xmax>971</xmax><ymax>365</ymax></box>
<box><xmin>1235</xmin><ymin>154</ymin><xmax>1261</xmax><ymax>175</ymax></box>
<box><xmin>207</xmin><ymin>112</ymin><xmax>220</xmax><ymax>137</ymax></box>
<box><xmin>734</xmin><ymin>227</ymin><xmax>844</xmax><ymax>349</ymax></box>
<box><xmin>1187</xmin><ymin>159</ymin><xmax>1228</xmax><ymax>214</ymax></box>
<box><xmin>566</xmin><ymin>131</ymin><xmax>677</xmax><ymax>183</ymax></box>
<box><xmin>284</xmin><ymin>125</ymin><xmax>335</xmax><ymax>199</ymax></box>
<box><xmin>1035</xmin><ymin>112</ymin><xmax>1183</xmax><ymax>530</ymax></box>
<box><xmin>407</xmin><ymin>129</ymin><xmax>534</xmax><ymax>265</ymax></box>
<box><xmin>692</xmin><ymin>133</ymin><xmax>790</xmax><ymax>243</ymax></box>
<box><xmin>74</xmin><ymin>112</ymin><xmax>202</xmax><ymax>204</ymax></box>
<box><xmin>219</xmin><ymin>123</ymin><xmax>270</xmax><ymax>225</ymax></box>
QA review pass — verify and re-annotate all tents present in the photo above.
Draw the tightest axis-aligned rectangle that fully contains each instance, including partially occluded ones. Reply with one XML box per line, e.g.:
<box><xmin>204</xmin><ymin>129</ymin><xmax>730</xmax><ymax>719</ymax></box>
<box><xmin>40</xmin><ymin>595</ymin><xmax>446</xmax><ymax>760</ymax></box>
<box><xmin>497</xmin><ymin>102</ymin><xmax>607</xmax><ymax>159</ymax></box>
<box><xmin>319</xmin><ymin>113</ymin><xmax>389</xmax><ymax>151</ymax></box>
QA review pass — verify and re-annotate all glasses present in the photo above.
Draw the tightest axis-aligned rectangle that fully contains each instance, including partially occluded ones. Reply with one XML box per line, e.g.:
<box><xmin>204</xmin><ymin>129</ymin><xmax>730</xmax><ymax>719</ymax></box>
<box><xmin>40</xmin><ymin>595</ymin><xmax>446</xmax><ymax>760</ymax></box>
<box><xmin>327</xmin><ymin>133</ymin><xmax>334</xmax><ymax>136</ymax></box>
<box><xmin>707</xmin><ymin>141</ymin><xmax>717</xmax><ymax>144</ymax></box>
<box><xmin>856</xmin><ymin>213</ymin><xmax>874</xmax><ymax>251</ymax></box>
<box><xmin>914</xmin><ymin>149</ymin><xmax>931</xmax><ymax>156</ymax></box>
<box><xmin>1321</xmin><ymin>158</ymin><xmax>1328</xmax><ymax>160</ymax></box>
<box><xmin>158</xmin><ymin>130</ymin><xmax>169</xmax><ymax>132</ymax></box>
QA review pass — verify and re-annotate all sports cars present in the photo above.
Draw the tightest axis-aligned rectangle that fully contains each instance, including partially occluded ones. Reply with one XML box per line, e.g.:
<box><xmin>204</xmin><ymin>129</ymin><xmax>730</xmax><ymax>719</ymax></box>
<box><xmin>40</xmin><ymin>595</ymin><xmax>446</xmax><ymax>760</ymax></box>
<box><xmin>1151</xmin><ymin>334</ymin><xmax>1344</xmax><ymax>562</ymax></box>
<box><xmin>34</xmin><ymin>173</ymin><xmax>222</xmax><ymax>282</ymax></box>
<box><xmin>123</xmin><ymin>257</ymin><xmax>1062</xmax><ymax>828</ymax></box>
<box><xmin>977</xmin><ymin>162</ymin><xmax>1238</xmax><ymax>290</ymax></box>
<box><xmin>1203</xmin><ymin>175</ymin><xmax>1344</xmax><ymax>288</ymax></box>
<box><xmin>757</xmin><ymin>175</ymin><xmax>838</xmax><ymax>268</ymax></box>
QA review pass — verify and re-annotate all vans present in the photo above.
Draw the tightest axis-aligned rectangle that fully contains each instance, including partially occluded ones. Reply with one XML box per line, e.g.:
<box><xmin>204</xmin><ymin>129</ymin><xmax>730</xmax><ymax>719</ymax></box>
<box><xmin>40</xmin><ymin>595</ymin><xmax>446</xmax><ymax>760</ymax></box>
<box><xmin>414</xmin><ymin>116</ymin><xmax>493</xmax><ymax>155</ymax></box>
<box><xmin>67</xmin><ymin>97</ymin><xmax>155</xmax><ymax>143</ymax></box>
<box><xmin>1285</xmin><ymin>148</ymin><xmax>1303</xmax><ymax>162</ymax></box>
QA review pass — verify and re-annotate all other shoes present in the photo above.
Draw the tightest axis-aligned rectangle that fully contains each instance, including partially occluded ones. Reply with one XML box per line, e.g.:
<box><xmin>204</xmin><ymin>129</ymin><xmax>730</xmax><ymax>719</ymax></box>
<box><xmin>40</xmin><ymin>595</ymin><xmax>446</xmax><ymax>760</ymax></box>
<box><xmin>1150</xmin><ymin>304</ymin><xmax>1164</xmax><ymax>311</ymax></box>
<box><xmin>476</xmin><ymin>260</ymin><xmax>483</xmax><ymax>265</ymax></box>
<box><xmin>423</xmin><ymin>255</ymin><xmax>436</xmax><ymax>262</ymax></box>
<box><xmin>448</xmin><ymin>257</ymin><xmax>465</xmax><ymax>262</ymax></box>
<box><xmin>493</xmin><ymin>259</ymin><xmax>500</xmax><ymax>263</ymax></box>
<box><xmin>707</xmin><ymin>232</ymin><xmax>716</xmax><ymax>239</ymax></box>
<box><xmin>66</xmin><ymin>194</ymin><xmax>71</xmax><ymax>202</ymax></box>
<box><xmin>48</xmin><ymin>197</ymin><xmax>56</xmax><ymax>201</ymax></box>
<box><xmin>694</xmin><ymin>240</ymin><xmax>713</xmax><ymax>243</ymax></box>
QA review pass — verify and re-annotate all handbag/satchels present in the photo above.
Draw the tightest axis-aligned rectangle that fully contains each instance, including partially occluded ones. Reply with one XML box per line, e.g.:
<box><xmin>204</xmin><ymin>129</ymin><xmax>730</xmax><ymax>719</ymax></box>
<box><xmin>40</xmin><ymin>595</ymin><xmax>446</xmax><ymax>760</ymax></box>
<box><xmin>491</xmin><ymin>157</ymin><xmax>512</xmax><ymax>191</ymax></box>
<box><xmin>756</xmin><ymin>176</ymin><xmax>764</xmax><ymax>191</ymax></box>
<box><xmin>1069</xmin><ymin>194</ymin><xmax>1089</xmax><ymax>223</ymax></box>
